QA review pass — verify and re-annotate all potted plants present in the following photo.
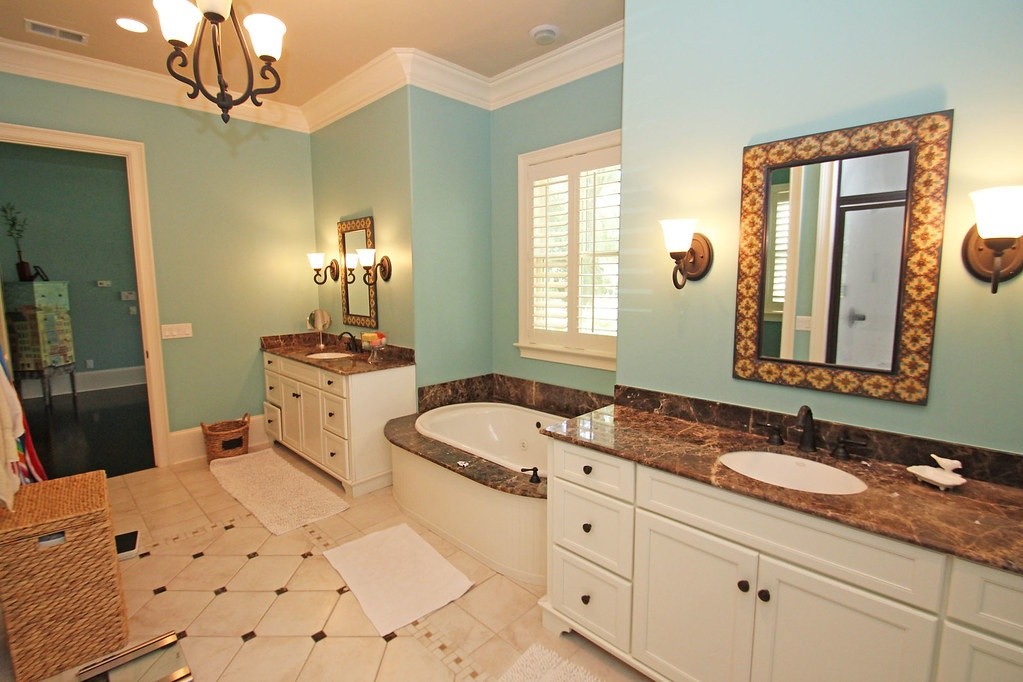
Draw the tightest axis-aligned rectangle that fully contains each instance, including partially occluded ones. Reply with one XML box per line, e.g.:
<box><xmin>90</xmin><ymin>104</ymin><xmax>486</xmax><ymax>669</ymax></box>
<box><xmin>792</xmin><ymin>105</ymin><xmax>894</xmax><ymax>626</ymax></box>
<box><xmin>0</xmin><ymin>201</ymin><xmax>34</xmax><ymax>281</ymax></box>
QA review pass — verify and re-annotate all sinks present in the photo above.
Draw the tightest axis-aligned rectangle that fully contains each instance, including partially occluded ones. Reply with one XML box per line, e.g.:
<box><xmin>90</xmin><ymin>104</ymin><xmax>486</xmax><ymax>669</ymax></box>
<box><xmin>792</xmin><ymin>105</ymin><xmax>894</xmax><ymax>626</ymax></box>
<box><xmin>718</xmin><ymin>449</ymin><xmax>869</xmax><ymax>496</ymax></box>
<box><xmin>306</xmin><ymin>352</ymin><xmax>354</xmax><ymax>359</ymax></box>
<box><xmin>414</xmin><ymin>401</ymin><xmax>573</xmax><ymax>479</ymax></box>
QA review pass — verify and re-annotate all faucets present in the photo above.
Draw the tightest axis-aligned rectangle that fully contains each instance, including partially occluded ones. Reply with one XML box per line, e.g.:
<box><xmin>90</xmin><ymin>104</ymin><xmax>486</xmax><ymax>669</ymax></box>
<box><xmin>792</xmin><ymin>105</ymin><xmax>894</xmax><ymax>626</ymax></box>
<box><xmin>792</xmin><ymin>404</ymin><xmax>818</xmax><ymax>452</ymax></box>
<box><xmin>337</xmin><ymin>331</ymin><xmax>356</xmax><ymax>353</ymax></box>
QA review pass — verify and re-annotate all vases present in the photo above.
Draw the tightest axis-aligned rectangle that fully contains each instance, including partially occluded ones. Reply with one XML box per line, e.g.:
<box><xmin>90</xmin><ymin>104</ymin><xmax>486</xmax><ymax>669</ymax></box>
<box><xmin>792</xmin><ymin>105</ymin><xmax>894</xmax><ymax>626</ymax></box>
<box><xmin>368</xmin><ymin>348</ymin><xmax>387</xmax><ymax>366</ymax></box>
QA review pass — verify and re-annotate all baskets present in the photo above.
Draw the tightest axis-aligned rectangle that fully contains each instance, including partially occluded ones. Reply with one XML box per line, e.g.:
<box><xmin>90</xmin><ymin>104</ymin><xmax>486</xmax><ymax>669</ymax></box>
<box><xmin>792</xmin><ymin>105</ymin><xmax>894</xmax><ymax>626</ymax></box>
<box><xmin>200</xmin><ymin>413</ymin><xmax>250</xmax><ymax>465</ymax></box>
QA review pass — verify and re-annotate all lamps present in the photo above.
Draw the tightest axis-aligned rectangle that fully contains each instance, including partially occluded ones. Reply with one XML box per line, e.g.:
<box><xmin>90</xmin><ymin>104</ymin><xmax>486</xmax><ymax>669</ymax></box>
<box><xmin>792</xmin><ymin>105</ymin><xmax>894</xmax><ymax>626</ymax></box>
<box><xmin>356</xmin><ymin>249</ymin><xmax>392</xmax><ymax>285</ymax></box>
<box><xmin>152</xmin><ymin>0</ymin><xmax>288</xmax><ymax>125</ymax></box>
<box><xmin>659</xmin><ymin>217</ymin><xmax>714</xmax><ymax>290</ymax></box>
<box><xmin>307</xmin><ymin>252</ymin><xmax>339</xmax><ymax>285</ymax></box>
<box><xmin>730</xmin><ymin>110</ymin><xmax>954</xmax><ymax>406</ymax></box>
<box><xmin>961</xmin><ymin>186</ymin><xmax>1023</xmax><ymax>294</ymax></box>
<box><xmin>346</xmin><ymin>253</ymin><xmax>358</xmax><ymax>284</ymax></box>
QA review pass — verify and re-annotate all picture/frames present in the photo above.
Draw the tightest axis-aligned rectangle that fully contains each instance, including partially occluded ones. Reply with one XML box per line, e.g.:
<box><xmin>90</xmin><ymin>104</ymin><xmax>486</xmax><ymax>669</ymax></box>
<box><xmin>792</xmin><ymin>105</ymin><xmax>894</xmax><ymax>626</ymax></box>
<box><xmin>33</xmin><ymin>265</ymin><xmax>50</xmax><ymax>281</ymax></box>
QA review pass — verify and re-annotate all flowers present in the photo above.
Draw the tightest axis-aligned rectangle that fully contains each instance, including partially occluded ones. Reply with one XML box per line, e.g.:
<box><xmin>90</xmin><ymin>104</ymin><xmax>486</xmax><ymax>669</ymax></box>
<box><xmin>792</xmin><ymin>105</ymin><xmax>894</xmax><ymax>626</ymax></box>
<box><xmin>362</xmin><ymin>332</ymin><xmax>387</xmax><ymax>350</ymax></box>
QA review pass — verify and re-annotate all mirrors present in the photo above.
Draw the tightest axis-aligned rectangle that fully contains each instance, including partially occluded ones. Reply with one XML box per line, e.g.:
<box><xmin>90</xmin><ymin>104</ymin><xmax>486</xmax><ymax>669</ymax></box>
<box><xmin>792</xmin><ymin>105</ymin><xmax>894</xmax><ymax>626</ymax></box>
<box><xmin>337</xmin><ymin>216</ymin><xmax>379</xmax><ymax>329</ymax></box>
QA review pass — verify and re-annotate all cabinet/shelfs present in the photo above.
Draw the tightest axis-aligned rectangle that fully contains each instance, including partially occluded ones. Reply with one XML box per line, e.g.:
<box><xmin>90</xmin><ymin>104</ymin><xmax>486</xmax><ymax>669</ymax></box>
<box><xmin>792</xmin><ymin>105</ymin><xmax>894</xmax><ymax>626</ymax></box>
<box><xmin>262</xmin><ymin>351</ymin><xmax>416</xmax><ymax>498</ymax></box>
<box><xmin>537</xmin><ymin>436</ymin><xmax>1023</xmax><ymax>682</ymax></box>
<box><xmin>1</xmin><ymin>280</ymin><xmax>76</xmax><ymax>405</ymax></box>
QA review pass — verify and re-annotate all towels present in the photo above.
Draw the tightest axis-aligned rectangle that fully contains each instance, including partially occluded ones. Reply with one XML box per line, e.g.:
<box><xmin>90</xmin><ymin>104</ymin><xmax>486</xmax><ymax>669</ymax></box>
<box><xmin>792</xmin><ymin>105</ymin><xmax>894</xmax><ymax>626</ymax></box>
<box><xmin>0</xmin><ymin>358</ymin><xmax>27</xmax><ymax>514</ymax></box>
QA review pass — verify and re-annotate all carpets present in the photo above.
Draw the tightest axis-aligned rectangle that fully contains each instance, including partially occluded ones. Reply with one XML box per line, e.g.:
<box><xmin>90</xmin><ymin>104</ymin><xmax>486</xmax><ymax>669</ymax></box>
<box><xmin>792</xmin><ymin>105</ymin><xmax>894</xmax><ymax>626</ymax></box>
<box><xmin>209</xmin><ymin>448</ymin><xmax>350</xmax><ymax>537</ymax></box>
<box><xmin>323</xmin><ymin>522</ymin><xmax>476</xmax><ymax>636</ymax></box>
